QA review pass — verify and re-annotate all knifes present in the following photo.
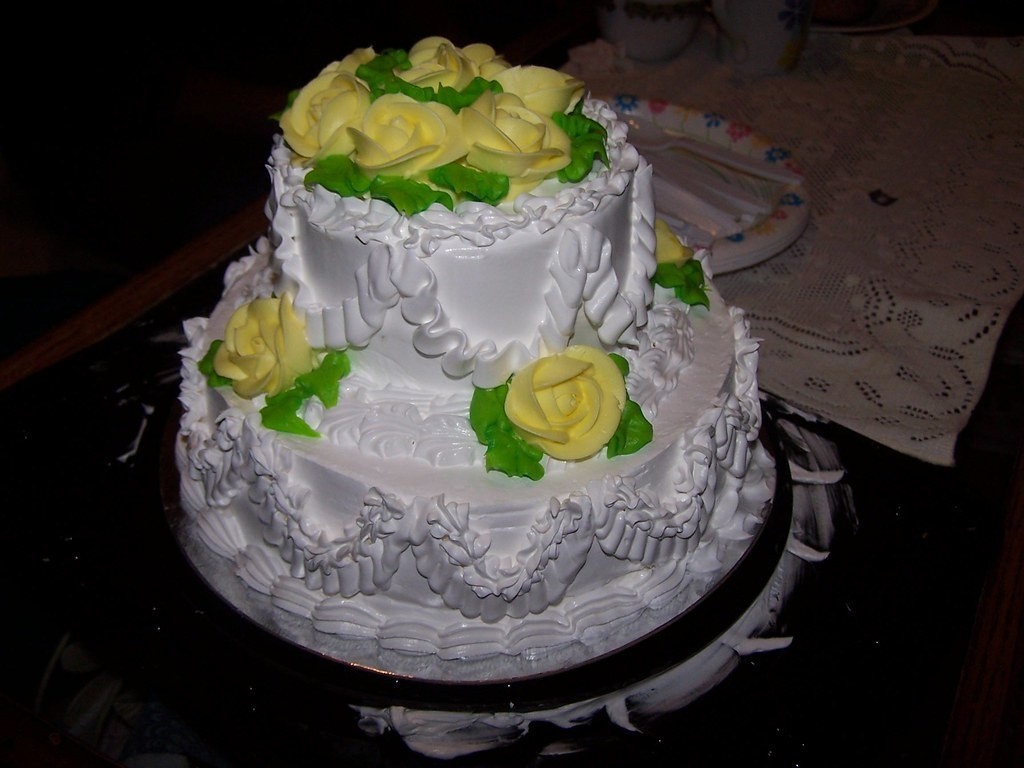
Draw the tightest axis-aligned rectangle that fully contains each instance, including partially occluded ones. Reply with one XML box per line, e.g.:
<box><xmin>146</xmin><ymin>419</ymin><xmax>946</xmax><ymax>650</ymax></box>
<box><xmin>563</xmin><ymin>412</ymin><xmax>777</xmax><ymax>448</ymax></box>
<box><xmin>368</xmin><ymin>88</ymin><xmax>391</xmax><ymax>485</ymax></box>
<box><xmin>617</xmin><ymin>112</ymin><xmax>804</xmax><ymax>187</ymax></box>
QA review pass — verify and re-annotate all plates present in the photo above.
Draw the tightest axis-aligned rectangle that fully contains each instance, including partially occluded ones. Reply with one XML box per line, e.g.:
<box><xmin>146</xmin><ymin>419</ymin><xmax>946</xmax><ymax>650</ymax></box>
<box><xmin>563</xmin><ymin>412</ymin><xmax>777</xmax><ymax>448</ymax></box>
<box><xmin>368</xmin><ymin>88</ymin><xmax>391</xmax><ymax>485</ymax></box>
<box><xmin>600</xmin><ymin>98</ymin><xmax>812</xmax><ymax>274</ymax></box>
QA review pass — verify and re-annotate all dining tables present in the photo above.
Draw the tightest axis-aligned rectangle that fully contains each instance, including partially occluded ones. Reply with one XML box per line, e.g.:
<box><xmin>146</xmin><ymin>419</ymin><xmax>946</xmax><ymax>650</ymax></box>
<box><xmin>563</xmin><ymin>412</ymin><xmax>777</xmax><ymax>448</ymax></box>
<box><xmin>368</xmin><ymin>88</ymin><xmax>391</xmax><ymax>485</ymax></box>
<box><xmin>1</xmin><ymin>0</ymin><xmax>1023</xmax><ymax>768</ymax></box>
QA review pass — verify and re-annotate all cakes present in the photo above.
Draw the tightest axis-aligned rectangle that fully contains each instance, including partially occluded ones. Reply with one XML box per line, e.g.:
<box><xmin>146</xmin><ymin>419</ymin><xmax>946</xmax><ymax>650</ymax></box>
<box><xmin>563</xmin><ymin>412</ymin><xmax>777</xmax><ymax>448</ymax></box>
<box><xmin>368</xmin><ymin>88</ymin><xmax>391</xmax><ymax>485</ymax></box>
<box><xmin>163</xmin><ymin>35</ymin><xmax>777</xmax><ymax>684</ymax></box>
